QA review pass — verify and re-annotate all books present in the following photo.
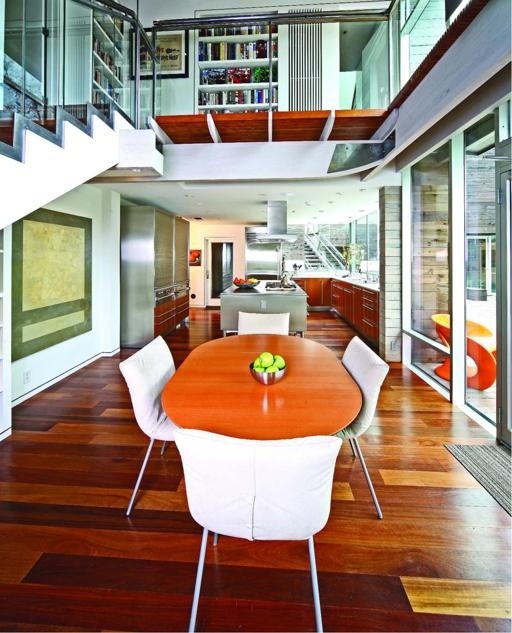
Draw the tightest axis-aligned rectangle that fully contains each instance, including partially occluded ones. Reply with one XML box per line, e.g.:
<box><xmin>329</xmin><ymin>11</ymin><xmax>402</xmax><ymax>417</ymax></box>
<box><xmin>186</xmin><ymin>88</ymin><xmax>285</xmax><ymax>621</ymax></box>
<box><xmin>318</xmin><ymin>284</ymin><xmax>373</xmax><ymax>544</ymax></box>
<box><xmin>196</xmin><ymin>23</ymin><xmax>279</xmax><ymax>113</ymax></box>
<box><xmin>91</xmin><ymin>9</ymin><xmax>124</xmax><ymax>117</ymax></box>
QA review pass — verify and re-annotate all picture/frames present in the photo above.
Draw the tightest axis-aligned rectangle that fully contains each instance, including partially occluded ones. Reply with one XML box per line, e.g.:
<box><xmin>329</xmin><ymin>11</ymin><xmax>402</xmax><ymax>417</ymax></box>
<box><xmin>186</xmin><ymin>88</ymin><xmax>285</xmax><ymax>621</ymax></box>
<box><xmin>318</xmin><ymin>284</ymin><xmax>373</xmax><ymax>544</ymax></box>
<box><xmin>128</xmin><ymin>28</ymin><xmax>189</xmax><ymax>80</ymax></box>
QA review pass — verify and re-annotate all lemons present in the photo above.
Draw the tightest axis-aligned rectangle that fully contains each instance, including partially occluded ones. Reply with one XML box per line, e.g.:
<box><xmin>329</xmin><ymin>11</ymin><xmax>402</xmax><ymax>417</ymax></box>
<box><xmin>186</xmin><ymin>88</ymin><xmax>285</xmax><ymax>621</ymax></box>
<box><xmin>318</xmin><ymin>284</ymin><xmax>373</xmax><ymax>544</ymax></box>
<box><xmin>247</xmin><ymin>279</ymin><xmax>257</xmax><ymax>283</ymax></box>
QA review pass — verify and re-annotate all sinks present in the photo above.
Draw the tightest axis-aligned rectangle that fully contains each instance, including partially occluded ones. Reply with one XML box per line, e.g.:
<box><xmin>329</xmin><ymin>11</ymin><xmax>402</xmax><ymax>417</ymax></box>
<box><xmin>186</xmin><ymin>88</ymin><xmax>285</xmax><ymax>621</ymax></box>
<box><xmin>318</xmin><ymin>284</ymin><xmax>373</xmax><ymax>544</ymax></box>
<box><xmin>265</xmin><ymin>280</ymin><xmax>297</xmax><ymax>291</ymax></box>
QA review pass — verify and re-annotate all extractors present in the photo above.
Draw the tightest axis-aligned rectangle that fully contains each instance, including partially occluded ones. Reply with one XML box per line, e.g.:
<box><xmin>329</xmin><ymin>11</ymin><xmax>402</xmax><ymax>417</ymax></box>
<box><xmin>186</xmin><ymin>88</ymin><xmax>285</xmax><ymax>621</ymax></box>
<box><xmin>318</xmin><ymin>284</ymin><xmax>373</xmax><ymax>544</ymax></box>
<box><xmin>255</xmin><ymin>199</ymin><xmax>299</xmax><ymax>243</ymax></box>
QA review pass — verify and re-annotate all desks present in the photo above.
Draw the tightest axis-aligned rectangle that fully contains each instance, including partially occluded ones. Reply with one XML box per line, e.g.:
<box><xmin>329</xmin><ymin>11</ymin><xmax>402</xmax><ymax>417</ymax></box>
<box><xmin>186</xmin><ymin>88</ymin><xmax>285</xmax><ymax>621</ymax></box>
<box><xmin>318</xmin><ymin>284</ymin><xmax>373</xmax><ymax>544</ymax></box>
<box><xmin>182</xmin><ymin>335</ymin><xmax>358</xmax><ymax>436</ymax></box>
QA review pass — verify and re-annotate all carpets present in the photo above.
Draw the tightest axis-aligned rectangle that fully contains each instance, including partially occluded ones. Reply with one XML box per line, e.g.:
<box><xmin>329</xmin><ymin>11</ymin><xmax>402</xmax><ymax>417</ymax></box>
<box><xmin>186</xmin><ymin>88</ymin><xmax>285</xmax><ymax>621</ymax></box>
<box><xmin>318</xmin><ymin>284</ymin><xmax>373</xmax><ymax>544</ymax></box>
<box><xmin>443</xmin><ymin>443</ymin><xmax>511</xmax><ymax>518</ymax></box>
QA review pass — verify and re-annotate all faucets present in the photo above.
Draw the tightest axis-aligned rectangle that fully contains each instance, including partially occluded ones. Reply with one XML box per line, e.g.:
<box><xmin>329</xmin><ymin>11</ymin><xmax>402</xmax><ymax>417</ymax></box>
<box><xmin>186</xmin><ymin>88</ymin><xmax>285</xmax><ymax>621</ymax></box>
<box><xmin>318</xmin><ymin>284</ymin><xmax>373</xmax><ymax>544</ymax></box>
<box><xmin>280</xmin><ymin>254</ymin><xmax>286</xmax><ymax>277</ymax></box>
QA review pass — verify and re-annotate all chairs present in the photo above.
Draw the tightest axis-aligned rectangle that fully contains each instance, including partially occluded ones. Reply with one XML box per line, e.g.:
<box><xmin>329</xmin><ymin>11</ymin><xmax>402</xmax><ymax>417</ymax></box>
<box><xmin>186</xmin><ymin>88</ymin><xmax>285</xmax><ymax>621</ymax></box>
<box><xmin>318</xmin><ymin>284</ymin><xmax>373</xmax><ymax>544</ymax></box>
<box><xmin>229</xmin><ymin>312</ymin><xmax>296</xmax><ymax>339</ymax></box>
<box><xmin>123</xmin><ymin>340</ymin><xmax>171</xmax><ymax>516</ymax></box>
<box><xmin>347</xmin><ymin>336</ymin><xmax>391</xmax><ymax>525</ymax></box>
<box><xmin>173</xmin><ymin>431</ymin><xmax>330</xmax><ymax>624</ymax></box>
<box><xmin>430</xmin><ymin>312</ymin><xmax>497</xmax><ymax>392</ymax></box>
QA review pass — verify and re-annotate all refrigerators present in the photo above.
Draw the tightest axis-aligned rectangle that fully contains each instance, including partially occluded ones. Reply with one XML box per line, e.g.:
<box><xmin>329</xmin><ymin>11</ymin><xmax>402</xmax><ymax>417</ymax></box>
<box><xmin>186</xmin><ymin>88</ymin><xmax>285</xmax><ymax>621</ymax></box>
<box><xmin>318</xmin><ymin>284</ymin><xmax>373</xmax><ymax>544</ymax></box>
<box><xmin>121</xmin><ymin>204</ymin><xmax>190</xmax><ymax>348</ymax></box>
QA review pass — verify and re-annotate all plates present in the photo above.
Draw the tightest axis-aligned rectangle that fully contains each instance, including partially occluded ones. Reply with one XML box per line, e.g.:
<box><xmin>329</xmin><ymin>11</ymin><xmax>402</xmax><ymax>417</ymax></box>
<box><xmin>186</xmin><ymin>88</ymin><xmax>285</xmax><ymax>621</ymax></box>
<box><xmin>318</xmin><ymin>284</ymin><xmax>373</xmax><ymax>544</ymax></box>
<box><xmin>233</xmin><ymin>280</ymin><xmax>261</xmax><ymax>290</ymax></box>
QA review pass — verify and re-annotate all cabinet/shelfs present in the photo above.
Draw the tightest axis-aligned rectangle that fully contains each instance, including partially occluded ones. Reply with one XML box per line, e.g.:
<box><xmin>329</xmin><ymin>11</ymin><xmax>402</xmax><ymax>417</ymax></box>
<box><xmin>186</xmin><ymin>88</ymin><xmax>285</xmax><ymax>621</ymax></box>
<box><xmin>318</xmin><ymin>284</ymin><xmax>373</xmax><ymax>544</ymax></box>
<box><xmin>63</xmin><ymin>0</ymin><xmax>130</xmax><ymax>118</ymax></box>
<box><xmin>294</xmin><ymin>278</ymin><xmax>332</xmax><ymax>306</ymax></box>
<box><xmin>330</xmin><ymin>279</ymin><xmax>378</xmax><ymax>344</ymax></box>
<box><xmin>193</xmin><ymin>6</ymin><xmax>277</xmax><ymax>115</ymax></box>
<box><xmin>154</xmin><ymin>295</ymin><xmax>189</xmax><ymax>338</ymax></box>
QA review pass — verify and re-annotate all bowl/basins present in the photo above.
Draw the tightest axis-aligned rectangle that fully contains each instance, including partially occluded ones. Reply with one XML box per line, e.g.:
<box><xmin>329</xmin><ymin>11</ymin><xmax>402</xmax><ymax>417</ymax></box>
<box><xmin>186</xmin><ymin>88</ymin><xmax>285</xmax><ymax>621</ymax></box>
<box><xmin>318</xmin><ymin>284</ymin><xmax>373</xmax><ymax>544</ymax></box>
<box><xmin>249</xmin><ymin>361</ymin><xmax>287</xmax><ymax>385</ymax></box>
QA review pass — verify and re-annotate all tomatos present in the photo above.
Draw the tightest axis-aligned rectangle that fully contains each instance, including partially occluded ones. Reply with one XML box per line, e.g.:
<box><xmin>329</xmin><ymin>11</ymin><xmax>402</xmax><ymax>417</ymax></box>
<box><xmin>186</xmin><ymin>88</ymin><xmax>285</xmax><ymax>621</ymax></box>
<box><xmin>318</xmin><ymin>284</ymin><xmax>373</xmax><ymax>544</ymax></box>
<box><xmin>190</xmin><ymin>250</ymin><xmax>200</xmax><ymax>262</ymax></box>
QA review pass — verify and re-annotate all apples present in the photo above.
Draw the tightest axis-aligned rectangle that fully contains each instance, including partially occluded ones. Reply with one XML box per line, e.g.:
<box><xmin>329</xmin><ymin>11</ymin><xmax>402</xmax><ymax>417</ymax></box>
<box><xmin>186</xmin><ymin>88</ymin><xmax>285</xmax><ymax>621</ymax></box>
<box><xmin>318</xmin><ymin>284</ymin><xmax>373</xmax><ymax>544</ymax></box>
<box><xmin>234</xmin><ymin>277</ymin><xmax>244</xmax><ymax>284</ymax></box>
<box><xmin>252</xmin><ymin>352</ymin><xmax>286</xmax><ymax>374</ymax></box>
